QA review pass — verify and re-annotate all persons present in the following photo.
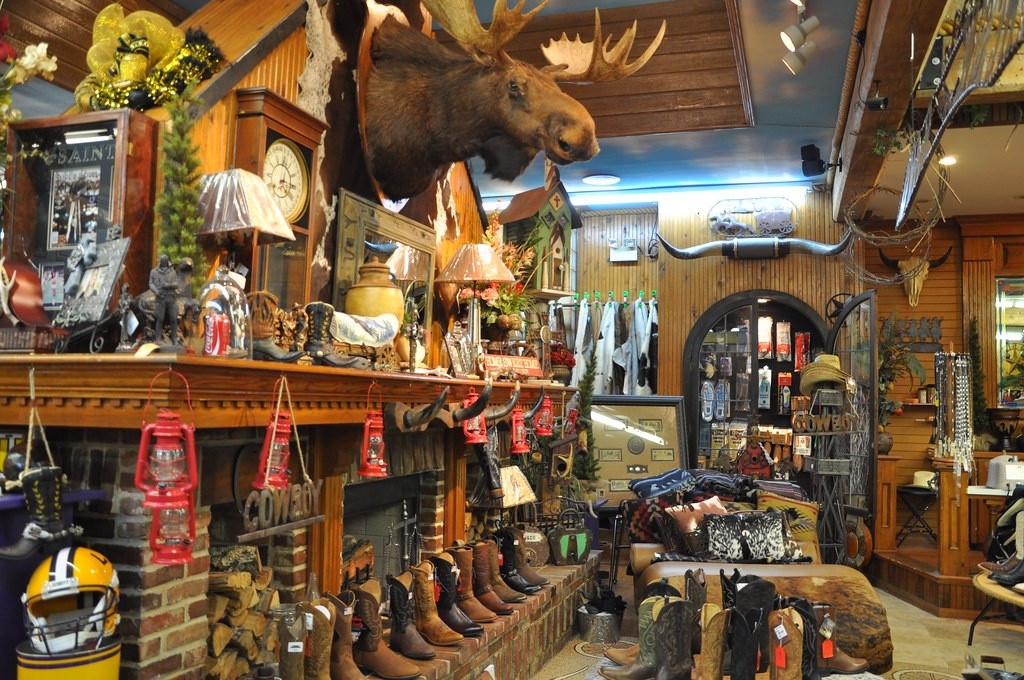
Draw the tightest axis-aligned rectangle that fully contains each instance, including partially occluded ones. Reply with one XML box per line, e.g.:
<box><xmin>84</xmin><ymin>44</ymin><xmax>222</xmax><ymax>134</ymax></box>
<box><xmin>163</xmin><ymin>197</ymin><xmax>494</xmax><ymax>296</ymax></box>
<box><xmin>149</xmin><ymin>255</ymin><xmax>180</xmax><ymax>347</ymax></box>
<box><xmin>291</xmin><ymin>302</ymin><xmax>308</xmax><ymax>340</ymax></box>
<box><xmin>49</xmin><ymin>273</ymin><xmax>58</xmax><ymax>306</ymax></box>
<box><xmin>78</xmin><ymin>268</ymin><xmax>102</xmax><ymax>298</ymax></box>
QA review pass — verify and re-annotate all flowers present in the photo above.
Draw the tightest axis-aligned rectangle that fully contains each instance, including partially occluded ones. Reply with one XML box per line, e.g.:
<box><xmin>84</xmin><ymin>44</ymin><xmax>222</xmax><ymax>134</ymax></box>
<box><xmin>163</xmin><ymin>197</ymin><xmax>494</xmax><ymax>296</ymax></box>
<box><xmin>460</xmin><ymin>196</ymin><xmax>556</xmax><ymax>348</ymax></box>
<box><xmin>0</xmin><ymin>14</ymin><xmax>59</xmax><ymax>209</ymax></box>
<box><xmin>539</xmin><ymin>343</ymin><xmax>576</xmax><ymax>368</ymax></box>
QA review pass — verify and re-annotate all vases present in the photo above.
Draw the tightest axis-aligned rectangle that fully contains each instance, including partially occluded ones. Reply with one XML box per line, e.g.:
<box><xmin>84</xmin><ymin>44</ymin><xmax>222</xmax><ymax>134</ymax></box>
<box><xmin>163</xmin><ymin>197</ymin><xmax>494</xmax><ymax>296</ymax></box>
<box><xmin>551</xmin><ymin>365</ymin><xmax>571</xmax><ymax>387</ymax></box>
<box><xmin>481</xmin><ymin>319</ymin><xmax>510</xmax><ymax>355</ymax></box>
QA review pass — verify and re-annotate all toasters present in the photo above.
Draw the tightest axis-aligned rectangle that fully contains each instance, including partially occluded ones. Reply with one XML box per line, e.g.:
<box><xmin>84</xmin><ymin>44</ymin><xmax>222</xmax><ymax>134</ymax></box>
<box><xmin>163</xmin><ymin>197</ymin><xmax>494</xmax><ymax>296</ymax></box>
<box><xmin>987</xmin><ymin>455</ymin><xmax>1018</xmax><ymax>491</ymax></box>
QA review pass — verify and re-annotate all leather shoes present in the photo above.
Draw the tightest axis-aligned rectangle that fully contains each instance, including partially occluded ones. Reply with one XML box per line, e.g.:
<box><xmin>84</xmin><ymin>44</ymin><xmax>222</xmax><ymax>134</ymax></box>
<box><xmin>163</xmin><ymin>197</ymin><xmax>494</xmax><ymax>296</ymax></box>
<box><xmin>977</xmin><ymin>551</ymin><xmax>1022</xmax><ymax>574</ymax></box>
<box><xmin>987</xmin><ymin>557</ymin><xmax>1024</xmax><ymax>585</ymax></box>
<box><xmin>252</xmin><ymin>334</ymin><xmax>306</xmax><ymax>364</ymax></box>
<box><xmin>311</xmin><ymin>351</ymin><xmax>360</xmax><ymax>369</ymax></box>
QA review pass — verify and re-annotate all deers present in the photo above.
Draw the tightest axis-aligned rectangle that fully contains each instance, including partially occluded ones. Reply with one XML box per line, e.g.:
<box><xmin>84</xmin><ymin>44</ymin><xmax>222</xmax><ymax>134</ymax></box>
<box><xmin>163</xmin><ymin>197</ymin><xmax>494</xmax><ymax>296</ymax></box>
<box><xmin>363</xmin><ymin>2</ymin><xmax>669</xmax><ymax>200</ymax></box>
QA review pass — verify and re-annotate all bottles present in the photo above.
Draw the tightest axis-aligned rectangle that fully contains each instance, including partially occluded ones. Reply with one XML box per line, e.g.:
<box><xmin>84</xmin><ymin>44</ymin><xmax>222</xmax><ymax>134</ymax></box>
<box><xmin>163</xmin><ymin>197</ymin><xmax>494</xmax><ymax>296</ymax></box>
<box><xmin>919</xmin><ymin>384</ymin><xmax>935</xmax><ymax>404</ymax></box>
<box><xmin>304</xmin><ymin>572</ymin><xmax>323</xmax><ymax>603</ymax></box>
<box><xmin>344</xmin><ymin>255</ymin><xmax>405</xmax><ymax>343</ymax></box>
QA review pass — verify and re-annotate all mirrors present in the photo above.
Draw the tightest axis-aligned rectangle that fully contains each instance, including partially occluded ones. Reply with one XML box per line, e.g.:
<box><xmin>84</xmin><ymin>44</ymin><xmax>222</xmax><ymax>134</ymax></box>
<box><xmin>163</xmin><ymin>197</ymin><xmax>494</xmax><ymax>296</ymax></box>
<box><xmin>332</xmin><ymin>188</ymin><xmax>437</xmax><ymax>355</ymax></box>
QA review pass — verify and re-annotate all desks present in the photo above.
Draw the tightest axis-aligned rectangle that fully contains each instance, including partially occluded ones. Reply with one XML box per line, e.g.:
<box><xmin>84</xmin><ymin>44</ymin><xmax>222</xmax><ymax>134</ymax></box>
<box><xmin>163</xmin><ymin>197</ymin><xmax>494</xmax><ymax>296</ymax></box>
<box><xmin>968</xmin><ymin>572</ymin><xmax>1024</xmax><ymax>646</ymax></box>
<box><xmin>896</xmin><ymin>486</ymin><xmax>939</xmax><ymax>548</ymax></box>
<box><xmin>965</xmin><ymin>485</ymin><xmax>1014</xmax><ymax>559</ymax></box>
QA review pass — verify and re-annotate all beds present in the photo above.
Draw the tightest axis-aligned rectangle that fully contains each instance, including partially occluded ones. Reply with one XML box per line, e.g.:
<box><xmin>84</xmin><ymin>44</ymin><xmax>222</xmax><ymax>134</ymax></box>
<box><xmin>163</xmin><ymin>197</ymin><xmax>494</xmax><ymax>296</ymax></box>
<box><xmin>633</xmin><ymin>561</ymin><xmax>893</xmax><ymax>675</ymax></box>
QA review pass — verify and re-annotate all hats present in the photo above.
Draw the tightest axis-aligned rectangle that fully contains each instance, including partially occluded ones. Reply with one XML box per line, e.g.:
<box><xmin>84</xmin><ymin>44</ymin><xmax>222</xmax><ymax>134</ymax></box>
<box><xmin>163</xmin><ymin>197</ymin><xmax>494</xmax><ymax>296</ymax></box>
<box><xmin>799</xmin><ymin>354</ymin><xmax>854</xmax><ymax>396</ymax></box>
<box><xmin>903</xmin><ymin>471</ymin><xmax>939</xmax><ymax>491</ymax></box>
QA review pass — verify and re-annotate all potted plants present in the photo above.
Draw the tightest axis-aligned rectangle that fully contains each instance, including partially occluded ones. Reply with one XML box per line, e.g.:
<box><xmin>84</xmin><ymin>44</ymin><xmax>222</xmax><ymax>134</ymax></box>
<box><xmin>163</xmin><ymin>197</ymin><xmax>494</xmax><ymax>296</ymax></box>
<box><xmin>560</xmin><ymin>340</ymin><xmax>609</xmax><ymax>550</ymax></box>
<box><xmin>853</xmin><ymin>337</ymin><xmax>927</xmax><ymax>455</ymax></box>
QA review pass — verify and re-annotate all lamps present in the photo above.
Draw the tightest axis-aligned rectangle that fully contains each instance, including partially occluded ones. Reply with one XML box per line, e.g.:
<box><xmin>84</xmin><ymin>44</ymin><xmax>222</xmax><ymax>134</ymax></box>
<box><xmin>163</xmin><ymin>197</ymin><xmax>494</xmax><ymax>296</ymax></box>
<box><xmin>582</xmin><ymin>174</ymin><xmax>620</xmax><ymax>185</ymax></box>
<box><xmin>133</xmin><ymin>369</ymin><xmax>198</xmax><ymax>509</ymax></box>
<box><xmin>252</xmin><ymin>379</ymin><xmax>291</xmax><ymax>492</ymax></box>
<box><xmin>197</xmin><ymin>167</ymin><xmax>296</xmax><ymax>267</ymax></box>
<box><xmin>800</xmin><ymin>143</ymin><xmax>842</xmax><ymax>177</ymax></box>
<box><xmin>564</xmin><ymin>398</ymin><xmax>580</xmax><ymax>435</ymax></box>
<box><xmin>534</xmin><ymin>393</ymin><xmax>554</xmax><ymax>436</ymax></box>
<box><xmin>148</xmin><ymin>491</ymin><xmax>196</xmax><ymax>564</ymax></box>
<box><xmin>510</xmin><ymin>391</ymin><xmax>531</xmax><ymax>453</ymax></box>
<box><xmin>780</xmin><ymin>0</ymin><xmax>820</xmax><ymax>77</ymax></box>
<box><xmin>435</xmin><ymin>243</ymin><xmax>515</xmax><ymax>355</ymax></box>
<box><xmin>357</xmin><ymin>383</ymin><xmax>389</xmax><ymax>478</ymax></box>
<box><xmin>462</xmin><ymin>387</ymin><xmax>489</xmax><ymax>444</ymax></box>
<box><xmin>608</xmin><ymin>237</ymin><xmax>638</xmax><ymax>261</ymax></box>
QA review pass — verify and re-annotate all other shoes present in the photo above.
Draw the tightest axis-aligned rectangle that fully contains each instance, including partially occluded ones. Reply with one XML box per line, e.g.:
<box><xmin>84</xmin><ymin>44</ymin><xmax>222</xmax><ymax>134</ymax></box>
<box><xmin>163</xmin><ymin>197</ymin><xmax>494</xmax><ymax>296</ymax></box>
<box><xmin>994</xmin><ymin>527</ymin><xmax>1016</xmax><ymax>546</ymax></box>
<box><xmin>992</xmin><ymin>484</ymin><xmax>1024</xmax><ymax>527</ymax></box>
<box><xmin>993</xmin><ymin>550</ymin><xmax>1015</xmax><ymax>564</ymax></box>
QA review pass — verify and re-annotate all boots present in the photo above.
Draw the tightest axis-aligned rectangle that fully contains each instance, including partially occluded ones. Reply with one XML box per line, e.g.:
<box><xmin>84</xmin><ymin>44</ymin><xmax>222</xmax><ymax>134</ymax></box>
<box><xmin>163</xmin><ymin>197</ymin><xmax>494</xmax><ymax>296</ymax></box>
<box><xmin>597</xmin><ymin>567</ymin><xmax>869</xmax><ymax>680</ymax></box>
<box><xmin>576</xmin><ymin>416</ymin><xmax>592</xmax><ymax>456</ymax></box>
<box><xmin>272</xmin><ymin>523</ymin><xmax>550</xmax><ymax>680</ymax></box>
<box><xmin>2</xmin><ymin>465</ymin><xmax>85</xmax><ymax>561</ymax></box>
<box><xmin>305</xmin><ymin>301</ymin><xmax>335</xmax><ymax>360</ymax></box>
<box><xmin>473</xmin><ymin>425</ymin><xmax>503</xmax><ymax>501</ymax></box>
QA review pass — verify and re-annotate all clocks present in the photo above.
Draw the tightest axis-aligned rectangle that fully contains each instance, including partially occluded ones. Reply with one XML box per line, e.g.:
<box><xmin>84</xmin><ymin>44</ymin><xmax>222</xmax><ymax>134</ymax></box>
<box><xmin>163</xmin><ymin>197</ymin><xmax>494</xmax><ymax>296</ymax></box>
<box><xmin>235</xmin><ymin>86</ymin><xmax>331</xmax><ymax>309</ymax></box>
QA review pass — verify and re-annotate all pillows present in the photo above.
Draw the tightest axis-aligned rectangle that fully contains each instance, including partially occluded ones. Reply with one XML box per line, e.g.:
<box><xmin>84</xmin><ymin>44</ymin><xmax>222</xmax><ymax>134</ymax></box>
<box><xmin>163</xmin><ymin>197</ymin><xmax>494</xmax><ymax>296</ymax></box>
<box><xmin>623</xmin><ymin>472</ymin><xmax>821</xmax><ymax>575</ymax></box>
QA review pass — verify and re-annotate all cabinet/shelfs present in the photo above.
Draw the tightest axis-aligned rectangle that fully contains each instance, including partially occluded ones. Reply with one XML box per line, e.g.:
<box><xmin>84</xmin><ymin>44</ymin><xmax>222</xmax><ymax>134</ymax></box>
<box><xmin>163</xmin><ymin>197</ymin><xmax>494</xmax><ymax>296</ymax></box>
<box><xmin>972</xmin><ymin>452</ymin><xmax>1023</xmax><ymax>544</ymax></box>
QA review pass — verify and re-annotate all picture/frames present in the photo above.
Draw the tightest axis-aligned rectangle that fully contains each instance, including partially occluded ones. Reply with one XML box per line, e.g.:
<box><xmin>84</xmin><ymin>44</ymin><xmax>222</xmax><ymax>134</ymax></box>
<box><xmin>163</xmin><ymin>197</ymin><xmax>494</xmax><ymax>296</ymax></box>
<box><xmin>38</xmin><ymin>261</ymin><xmax>65</xmax><ymax>311</ymax></box>
<box><xmin>52</xmin><ymin>236</ymin><xmax>132</xmax><ymax>325</ymax></box>
<box><xmin>589</xmin><ymin>396</ymin><xmax>691</xmax><ymax>512</ymax></box>
<box><xmin>995</xmin><ymin>277</ymin><xmax>1024</xmax><ymax>410</ymax></box>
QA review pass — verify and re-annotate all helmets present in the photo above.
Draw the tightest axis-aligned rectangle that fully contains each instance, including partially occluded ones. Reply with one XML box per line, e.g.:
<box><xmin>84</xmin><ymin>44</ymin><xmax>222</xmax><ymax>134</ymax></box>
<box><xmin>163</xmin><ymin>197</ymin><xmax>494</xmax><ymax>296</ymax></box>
<box><xmin>21</xmin><ymin>546</ymin><xmax>120</xmax><ymax>637</ymax></box>
<box><xmin>1</xmin><ymin>438</ymin><xmax>68</xmax><ymax>495</ymax></box>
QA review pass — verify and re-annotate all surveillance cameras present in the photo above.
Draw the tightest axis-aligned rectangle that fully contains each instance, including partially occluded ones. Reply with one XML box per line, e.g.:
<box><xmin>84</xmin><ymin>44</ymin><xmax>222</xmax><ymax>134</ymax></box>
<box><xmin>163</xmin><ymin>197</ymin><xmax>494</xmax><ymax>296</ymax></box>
<box><xmin>866</xmin><ymin>96</ymin><xmax>888</xmax><ymax>111</ymax></box>
